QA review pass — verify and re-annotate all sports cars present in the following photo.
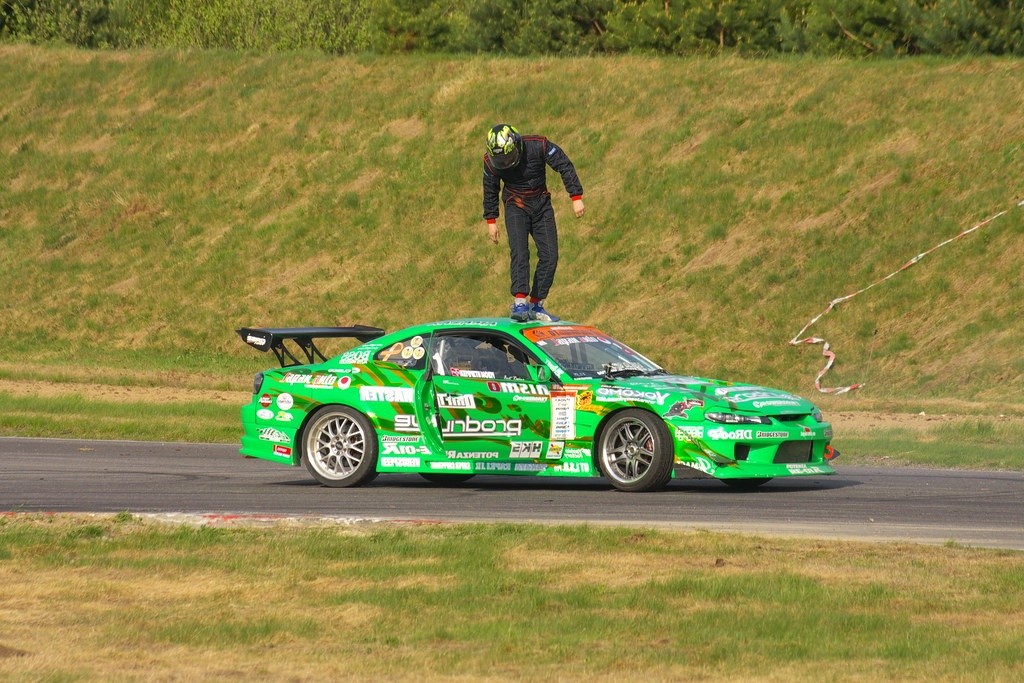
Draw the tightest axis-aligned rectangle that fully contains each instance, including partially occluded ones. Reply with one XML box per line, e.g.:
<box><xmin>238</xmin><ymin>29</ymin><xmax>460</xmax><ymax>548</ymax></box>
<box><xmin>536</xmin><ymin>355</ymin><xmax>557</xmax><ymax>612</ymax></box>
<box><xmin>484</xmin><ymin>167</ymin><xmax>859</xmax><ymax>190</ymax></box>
<box><xmin>236</xmin><ymin>317</ymin><xmax>840</xmax><ymax>493</ymax></box>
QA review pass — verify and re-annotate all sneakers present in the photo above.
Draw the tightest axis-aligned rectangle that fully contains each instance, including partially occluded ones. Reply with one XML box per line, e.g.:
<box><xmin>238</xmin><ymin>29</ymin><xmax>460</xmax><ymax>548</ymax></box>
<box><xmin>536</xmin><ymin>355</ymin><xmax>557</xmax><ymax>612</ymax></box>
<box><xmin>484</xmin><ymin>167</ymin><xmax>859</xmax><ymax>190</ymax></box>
<box><xmin>528</xmin><ymin>300</ymin><xmax>560</xmax><ymax>322</ymax></box>
<box><xmin>509</xmin><ymin>300</ymin><xmax>529</xmax><ymax>321</ymax></box>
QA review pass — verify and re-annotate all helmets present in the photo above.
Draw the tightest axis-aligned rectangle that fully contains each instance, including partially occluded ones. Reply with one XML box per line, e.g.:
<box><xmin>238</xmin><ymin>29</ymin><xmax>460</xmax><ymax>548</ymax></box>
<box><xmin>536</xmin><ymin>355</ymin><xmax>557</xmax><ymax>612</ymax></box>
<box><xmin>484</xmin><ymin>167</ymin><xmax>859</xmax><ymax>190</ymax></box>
<box><xmin>486</xmin><ymin>123</ymin><xmax>520</xmax><ymax>169</ymax></box>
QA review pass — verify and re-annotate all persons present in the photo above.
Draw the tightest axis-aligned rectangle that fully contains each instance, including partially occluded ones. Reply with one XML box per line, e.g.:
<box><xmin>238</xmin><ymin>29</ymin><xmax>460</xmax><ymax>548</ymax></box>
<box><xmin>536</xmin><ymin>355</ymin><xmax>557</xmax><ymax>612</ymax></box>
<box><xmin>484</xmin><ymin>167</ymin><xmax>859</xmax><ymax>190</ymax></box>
<box><xmin>483</xmin><ymin>124</ymin><xmax>585</xmax><ymax>322</ymax></box>
